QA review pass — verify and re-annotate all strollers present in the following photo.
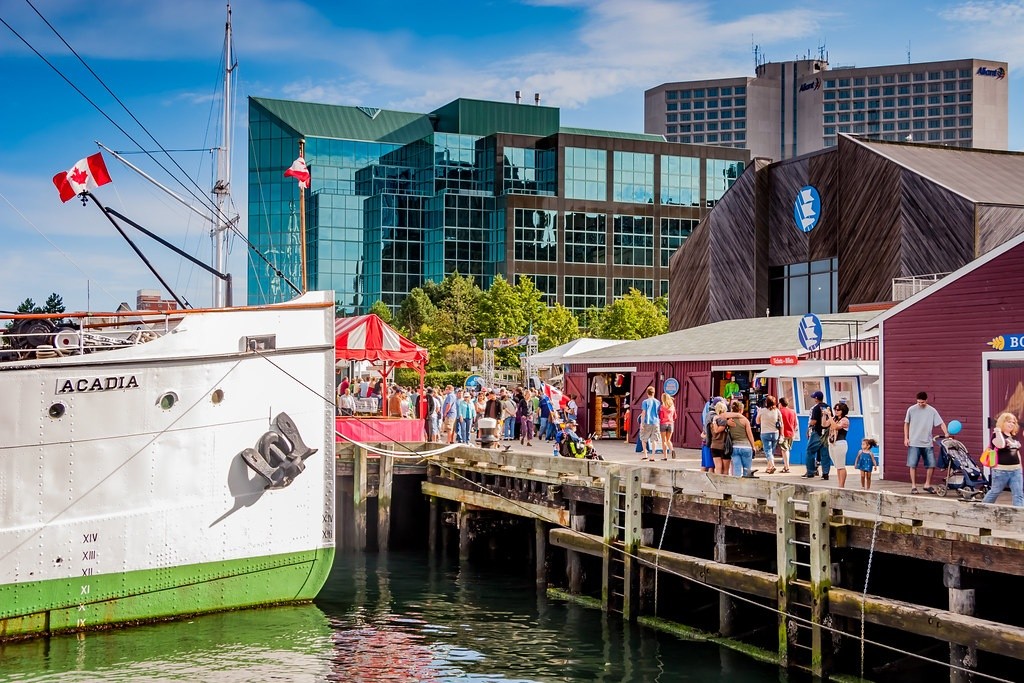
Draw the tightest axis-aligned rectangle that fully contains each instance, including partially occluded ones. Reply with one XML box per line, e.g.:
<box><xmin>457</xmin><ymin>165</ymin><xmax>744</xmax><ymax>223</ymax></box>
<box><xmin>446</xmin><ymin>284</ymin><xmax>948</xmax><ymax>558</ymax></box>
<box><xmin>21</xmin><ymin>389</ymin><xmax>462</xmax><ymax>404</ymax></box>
<box><xmin>933</xmin><ymin>435</ymin><xmax>992</xmax><ymax>502</ymax></box>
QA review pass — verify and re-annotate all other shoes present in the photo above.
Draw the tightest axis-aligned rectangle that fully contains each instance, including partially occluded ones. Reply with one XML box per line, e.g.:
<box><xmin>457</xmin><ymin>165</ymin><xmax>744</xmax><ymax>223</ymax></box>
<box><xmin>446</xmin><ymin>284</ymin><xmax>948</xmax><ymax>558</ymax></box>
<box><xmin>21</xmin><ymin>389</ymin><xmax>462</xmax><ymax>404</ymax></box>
<box><xmin>527</xmin><ymin>442</ymin><xmax>532</xmax><ymax>446</ymax></box>
<box><xmin>649</xmin><ymin>459</ymin><xmax>655</xmax><ymax>461</ymax></box>
<box><xmin>642</xmin><ymin>457</ymin><xmax>647</xmax><ymax>460</ymax></box>
<box><xmin>539</xmin><ymin>436</ymin><xmax>542</xmax><ymax>440</ymax></box>
<box><xmin>546</xmin><ymin>439</ymin><xmax>549</xmax><ymax>443</ymax></box>
<box><xmin>671</xmin><ymin>450</ymin><xmax>675</xmax><ymax>459</ymax></box>
<box><xmin>521</xmin><ymin>439</ymin><xmax>524</xmax><ymax>445</ymax></box>
<box><xmin>661</xmin><ymin>458</ymin><xmax>667</xmax><ymax>461</ymax></box>
<box><xmin>801</xmin><ymin>474</ymin><xmax>814</xmax><ymax>479</ymax></box>
<box><xmin>821</xmin><ymin>474</ymin><xmax>829</xmax><ymax>480</ymax></box>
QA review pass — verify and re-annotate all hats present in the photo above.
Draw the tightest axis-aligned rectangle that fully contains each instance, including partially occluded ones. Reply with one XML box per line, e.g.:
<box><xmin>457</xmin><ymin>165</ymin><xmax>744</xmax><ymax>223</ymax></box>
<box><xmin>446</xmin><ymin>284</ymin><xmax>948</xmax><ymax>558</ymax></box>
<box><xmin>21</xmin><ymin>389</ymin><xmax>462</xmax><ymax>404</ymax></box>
<box><xmin>566</xmin><ymin>419</ymin><xmax>578</xmax><ymax>426</ymax></box>
<box><xmin>713</xmin><ymin>396</ymin><xmax>730</xmax><ymax>406</ymax></box>
<box><xmin>811</xmin><ymin>391</ymin><xmax>823</xmax><ymax>401</ymax></box>
<box><xmin>464</xmin><ymin>392</ymin><xmax>470</xmax><ymax>398</ymax></box>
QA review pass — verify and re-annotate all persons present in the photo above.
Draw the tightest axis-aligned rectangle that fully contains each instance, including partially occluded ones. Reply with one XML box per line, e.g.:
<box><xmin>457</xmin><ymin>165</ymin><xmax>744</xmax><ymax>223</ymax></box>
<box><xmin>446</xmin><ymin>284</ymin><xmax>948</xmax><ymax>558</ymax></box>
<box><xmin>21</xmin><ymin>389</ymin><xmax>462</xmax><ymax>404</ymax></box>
<box><xmin>624</xmin><ymin>409</ymin><xmax>630</xmax><ymax>442</ymax></box>
<box><xmin>659</xmin><ymin>393</ymin><xmax>676</xmax><ymax>460</ymax></box>
<box><xmin>640</xmin><ymin>386</ymin><xmax>661</xmax><ymax>461</ymax></box>
<box><xmin>822</xmin><ymin>403</ymin><xmax>850</xmax><ymax>488</ymax></box>
<box><xmin>756</xmin><ymin>394</ymin><xmax>784</xmax><ymax>474</ymax></box>
<box><xmin>700</xmin><ymin>396</ymin><xmax>755</xmax><ymax>476</ymax></box>
<box><xmin>335</xmin><ymin>369</ymin><xmax>578</xmax><ymax>446</ymax></box>
<box><xmin>801</xmin><ymin>391</ymin><xmax>830</xmax><ymax>480</ymax></box>
<box><xmin>854</xmin><ymin>438</ymin><xmax>878</xmax><ymax>490</ymax></box>
<box><xmin>777</xmin><ymin>397</ymin><xmax>798</xmax><ymax>473</ymax></box>
<box><xmin>564</xmin><ymin>418</ymin><xmax>583</xmax><ymax>442</ymax></box>
<box><xmin>904</xmin><ymin>392</ymin><xmax>949</xmax><ymax>495</ymax></box>
<box><xmin>982</xmin><ymin>412</ymin><xmax>1023</xmax><ymax>507</ymax></box>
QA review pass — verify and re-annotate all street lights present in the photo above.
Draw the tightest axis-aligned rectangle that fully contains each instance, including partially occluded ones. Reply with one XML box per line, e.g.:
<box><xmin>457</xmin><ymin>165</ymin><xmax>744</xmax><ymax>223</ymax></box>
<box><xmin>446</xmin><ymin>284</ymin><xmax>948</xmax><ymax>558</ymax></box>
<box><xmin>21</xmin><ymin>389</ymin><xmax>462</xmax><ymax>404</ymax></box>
<box><xmin>469</xmin><ymin>336</ymin><xmax>479</xmax><ymax>375</ymax></box>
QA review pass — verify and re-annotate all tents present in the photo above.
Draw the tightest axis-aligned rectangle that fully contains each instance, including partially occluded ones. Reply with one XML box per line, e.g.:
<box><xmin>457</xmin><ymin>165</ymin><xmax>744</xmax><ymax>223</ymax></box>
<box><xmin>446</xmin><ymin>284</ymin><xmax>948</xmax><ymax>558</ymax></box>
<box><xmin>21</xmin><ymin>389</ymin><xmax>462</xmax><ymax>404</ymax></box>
<box><xmin>335</xmin><ymin>314</ymin><xmax>428</xmax><ymax>441</ymax></box>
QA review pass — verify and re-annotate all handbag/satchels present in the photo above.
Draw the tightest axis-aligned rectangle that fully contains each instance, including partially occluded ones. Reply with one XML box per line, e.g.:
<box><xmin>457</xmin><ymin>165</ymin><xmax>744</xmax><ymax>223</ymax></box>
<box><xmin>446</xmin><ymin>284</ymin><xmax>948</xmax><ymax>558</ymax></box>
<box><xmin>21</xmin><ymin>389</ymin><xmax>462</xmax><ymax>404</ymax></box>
<box><xmin>980</xmin><ymin>432</ymin><xmax>999</xmax><ymax>467</ymax></box>
<box><xmin>725</xmin><ymin>433</ymin><xmax>732</xmax><ymax>455</ymax></box>
<box><xmin>819</xmin><ymin>427</ymin><xmax>829</xmax><ymax>448</ymax></box>
<box><xmin>776</xmin><ymin>421</ymin><xmax>781</xmax><ymax>429</ymax></box>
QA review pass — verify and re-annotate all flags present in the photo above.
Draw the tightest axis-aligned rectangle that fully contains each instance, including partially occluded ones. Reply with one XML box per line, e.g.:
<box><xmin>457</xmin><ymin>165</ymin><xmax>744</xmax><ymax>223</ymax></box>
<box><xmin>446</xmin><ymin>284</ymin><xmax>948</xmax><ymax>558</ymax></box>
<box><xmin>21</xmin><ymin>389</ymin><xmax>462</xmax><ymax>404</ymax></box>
<box><xmin>543</xmin><ymin>383</ymin><xmax>570</xmax><ymax>409</ymax></box>
<box><xmin>53</xmin><ymin>152</ymin><xmax>112</xmax><ymax>203</ymax></box>
<box><xmin>284</xmin><ymin>157</ymin><xmax>310</xmax><ymax>189</ymax></box>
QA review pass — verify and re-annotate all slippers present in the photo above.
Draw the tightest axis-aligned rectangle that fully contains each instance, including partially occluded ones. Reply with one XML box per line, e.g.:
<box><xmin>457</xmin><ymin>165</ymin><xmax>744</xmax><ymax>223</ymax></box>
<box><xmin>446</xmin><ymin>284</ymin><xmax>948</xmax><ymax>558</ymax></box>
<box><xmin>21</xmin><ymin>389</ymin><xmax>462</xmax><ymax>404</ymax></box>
<box><xmin>923</xmin><ymin>487</ymin><xmax>936</xmax><ymax>494</ymax></box>
<box><xmin>911</xmin><ymin>488</ymin><xmax>919</xmax><ymax>494</ymax></box>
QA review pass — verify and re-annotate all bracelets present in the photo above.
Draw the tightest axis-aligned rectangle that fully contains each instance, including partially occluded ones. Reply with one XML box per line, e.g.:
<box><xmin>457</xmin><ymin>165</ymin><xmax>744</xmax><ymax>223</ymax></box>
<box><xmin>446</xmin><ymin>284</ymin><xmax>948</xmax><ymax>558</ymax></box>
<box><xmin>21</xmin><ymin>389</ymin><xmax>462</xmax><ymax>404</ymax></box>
<box><xmin>997</xmin><ymin>430</ymin><xmax>1001</xmax><ymax>432</ymax></box>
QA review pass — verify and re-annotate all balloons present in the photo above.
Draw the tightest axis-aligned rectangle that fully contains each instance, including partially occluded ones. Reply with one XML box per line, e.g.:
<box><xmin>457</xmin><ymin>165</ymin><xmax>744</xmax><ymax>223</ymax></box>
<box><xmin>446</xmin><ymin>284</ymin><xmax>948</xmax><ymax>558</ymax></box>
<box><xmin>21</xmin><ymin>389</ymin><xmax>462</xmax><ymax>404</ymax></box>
<box><xmin>948</xmin><ymin>420</ymin><xmax>962</xmax><ymax>435</ymax></box>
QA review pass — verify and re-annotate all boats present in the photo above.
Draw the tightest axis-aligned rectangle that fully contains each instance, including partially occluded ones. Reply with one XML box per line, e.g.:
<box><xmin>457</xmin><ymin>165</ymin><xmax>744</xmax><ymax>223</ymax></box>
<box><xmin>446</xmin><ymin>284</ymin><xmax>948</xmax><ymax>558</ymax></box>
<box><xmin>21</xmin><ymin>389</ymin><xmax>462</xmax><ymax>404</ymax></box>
<box><xmin>0</xmin><ymin>1</ymin><xmax>343</xmax><ymax>643</ymax></box>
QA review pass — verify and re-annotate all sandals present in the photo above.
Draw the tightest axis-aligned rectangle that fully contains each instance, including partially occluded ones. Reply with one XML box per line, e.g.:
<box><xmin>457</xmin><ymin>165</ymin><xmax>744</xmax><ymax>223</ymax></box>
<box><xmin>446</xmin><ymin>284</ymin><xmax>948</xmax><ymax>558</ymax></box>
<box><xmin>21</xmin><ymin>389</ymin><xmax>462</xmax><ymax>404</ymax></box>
<box><xmin>780</xmin><ymin>468</ymin><xmax>790</xmax><ymax>473</ymax></box>
<box><xmin>766</xmin><ymin>466</ymin><xmax>776</xmax><ymax>474</ymax></box>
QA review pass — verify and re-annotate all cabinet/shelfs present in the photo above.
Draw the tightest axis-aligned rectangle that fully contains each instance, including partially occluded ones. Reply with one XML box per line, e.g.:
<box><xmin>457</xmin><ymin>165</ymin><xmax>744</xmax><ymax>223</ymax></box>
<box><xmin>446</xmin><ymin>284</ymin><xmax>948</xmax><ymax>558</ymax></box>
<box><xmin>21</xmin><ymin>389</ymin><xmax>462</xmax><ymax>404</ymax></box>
<box><xmin>595</xmin><ymin>395</ymin><xmax>629</xmax><ymax>438</ymax></box>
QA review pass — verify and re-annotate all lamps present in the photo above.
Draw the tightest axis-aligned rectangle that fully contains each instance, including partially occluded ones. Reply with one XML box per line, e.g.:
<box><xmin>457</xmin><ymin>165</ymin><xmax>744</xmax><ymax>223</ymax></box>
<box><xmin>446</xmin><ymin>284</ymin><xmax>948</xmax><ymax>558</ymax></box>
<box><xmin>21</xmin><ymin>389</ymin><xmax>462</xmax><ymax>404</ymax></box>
<box><xmin>478</xmin><ymin>418</ymin><xmax>497</xmax><ymax>428</ymax></box>
<box><xmin>660</xmin><ymin>361</ymin><xmax>674</xmax><ymax>381</ymax></box>
